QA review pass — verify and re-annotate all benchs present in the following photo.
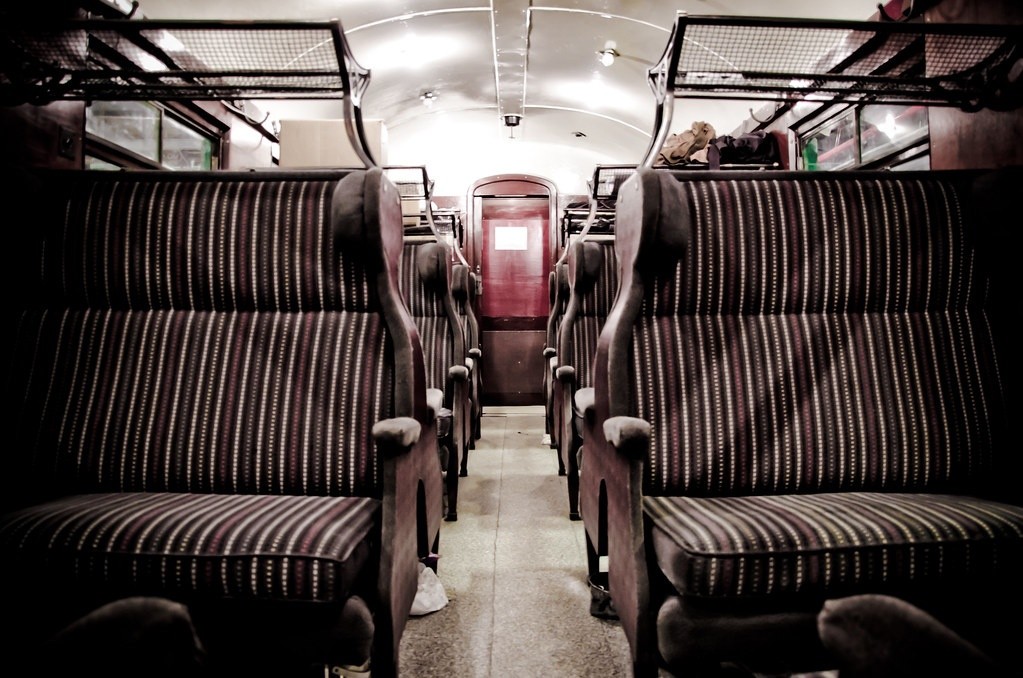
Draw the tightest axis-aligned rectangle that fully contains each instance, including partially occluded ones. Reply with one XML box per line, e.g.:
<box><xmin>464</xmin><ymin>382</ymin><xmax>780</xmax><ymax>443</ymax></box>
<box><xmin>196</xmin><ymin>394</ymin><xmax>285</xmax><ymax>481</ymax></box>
<box><xmin>1</xmin><ymin>165</ymin><xmax>485</xmax><ymax>677</ymax></box>
<box><xmin>541</xmin><ymin>162</ymin><xmax>1023</xmax><ymax>677</ymax></box>
